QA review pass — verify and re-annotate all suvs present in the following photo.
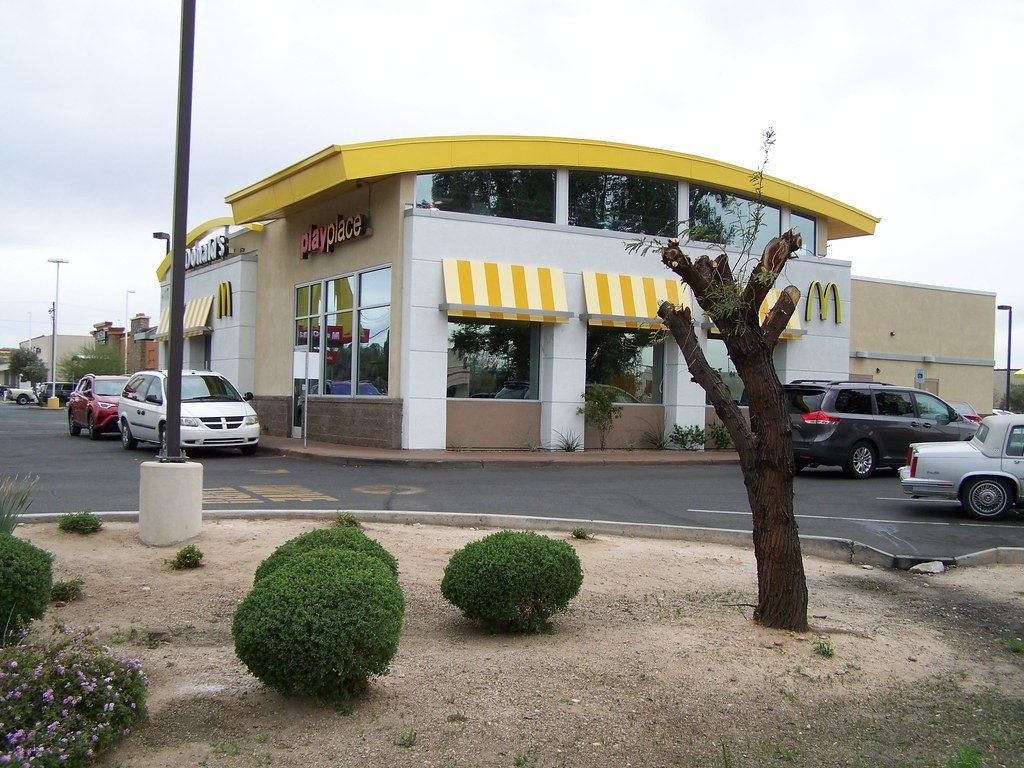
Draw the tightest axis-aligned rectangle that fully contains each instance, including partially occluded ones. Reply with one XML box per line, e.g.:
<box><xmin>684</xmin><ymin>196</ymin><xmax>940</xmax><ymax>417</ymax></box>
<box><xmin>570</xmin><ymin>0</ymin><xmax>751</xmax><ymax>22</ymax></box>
<box><xmin>68</xmin><ymin>373</ymin><xmax>133</xmax><ymax>440</ymax></box>
<box><xmin>781</xmin><ymin>379</ymin><xmax>984</xmax><ymax>479</ymax></box>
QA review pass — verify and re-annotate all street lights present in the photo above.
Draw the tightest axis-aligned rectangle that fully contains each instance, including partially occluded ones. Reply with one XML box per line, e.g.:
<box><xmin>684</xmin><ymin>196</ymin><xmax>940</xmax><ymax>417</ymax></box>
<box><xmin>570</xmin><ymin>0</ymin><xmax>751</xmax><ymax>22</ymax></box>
<box><xmin>48</xmin><ymin>257</ymin><xmax>70</xmax><ymax>396</ymax></box>
<box><xmin>122</xmin><ymin>289</ymin><xmax>138</xmax><ymax>377</ymax></box>
<box><xmin>997</xmin><ymin>305</ymin><xmax>1012</xmax><ymax>409</ymax></box>
<box><xmin>152</xmin><ymin>231</ymin><xmax>170</xmax><ymax>258</ymax></box>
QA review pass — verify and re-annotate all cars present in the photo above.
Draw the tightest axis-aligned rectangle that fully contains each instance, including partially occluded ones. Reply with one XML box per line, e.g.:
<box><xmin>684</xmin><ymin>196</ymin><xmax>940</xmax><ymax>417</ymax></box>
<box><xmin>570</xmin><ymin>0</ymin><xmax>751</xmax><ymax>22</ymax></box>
<box><xmin>994</xmin><ymin>408</ymin><xmax>1014</xmax><ymax>417</ymax></box>
<box><xmin>0</xmin><ymin>381</ymin><xmax>80</xmax><ymax>405</ymax></box>
<box><xmin>116</xmin><ymin>368</ymin><xmax>261</xmax><ymax>456</ymax></box>
<box><xmin>468</xmin><ymin>382</ymin><xmax>532</xmax><ymax>400</ymax></box>
<box><xmin>896</xmin><ymin>413</ymin><xmax>1024</xmax><ymax>520</ymax></box>
<box><xmin>586</xmin><ymin>383</ymin><xmax>641</xmax><ymax>403</ymax></box>
<box><xmin>296</xmin><ymin>379</ymin><xmax>383</xmax><ymax>429</ymax></box>
<box><xmin>934</xmin><ymin>399</ymin><xmax>983</xmax><ymax>425</ymax></box>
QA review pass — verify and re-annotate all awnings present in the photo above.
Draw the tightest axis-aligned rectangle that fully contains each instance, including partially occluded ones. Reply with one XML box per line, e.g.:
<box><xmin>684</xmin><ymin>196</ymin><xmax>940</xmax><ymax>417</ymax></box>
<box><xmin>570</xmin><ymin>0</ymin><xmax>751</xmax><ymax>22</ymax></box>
<box><xmin>443</xmin><ymin>259</ymin><xmax>807</xmax><ymax>340</ymax></box>
<box><xmin>154</xmin><ymin>296</ymin><xmax>214</xmax><ymax>342</ymax></box>
